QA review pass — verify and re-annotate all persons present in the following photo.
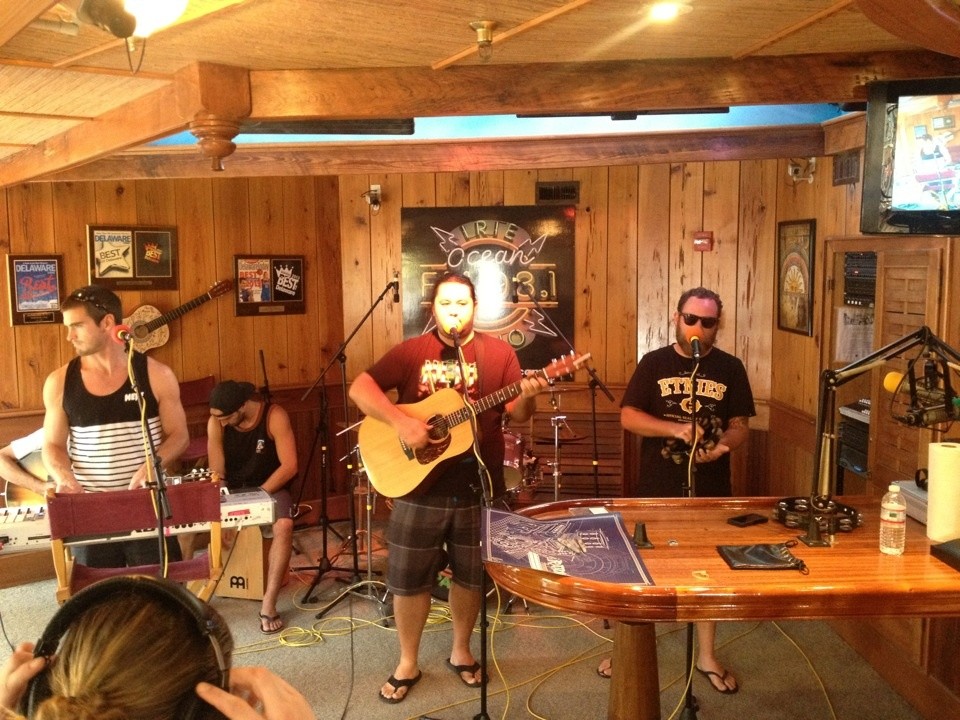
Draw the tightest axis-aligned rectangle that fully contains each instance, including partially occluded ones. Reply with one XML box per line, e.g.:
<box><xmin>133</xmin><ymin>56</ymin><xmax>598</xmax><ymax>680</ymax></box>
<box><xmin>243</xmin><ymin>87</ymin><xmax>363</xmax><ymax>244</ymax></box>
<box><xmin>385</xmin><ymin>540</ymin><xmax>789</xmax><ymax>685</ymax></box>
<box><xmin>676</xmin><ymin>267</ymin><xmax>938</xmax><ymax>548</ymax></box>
<box><xmin>40</xmin><ymin>285</ymin><xmax>188</xmax><ymax>588</ymax></box>
<box><xmin>179</xmin><ymin>380</ymin><xmax>298</xmax><ymax>634</ymax></box>
<box><xmin>0</xmin><ymin>428</ymin><xmax>61</xmax><ymax>499</ymax></box>
<box><xmin>349</xmin><ymin>271</ymin><xmax>548</xmax><ymax>703</ymax></box>
<box><xmin>1</xmin><ymin>574</ymin><xmax>316</xmax><ymax>720</ymax></box>
<box><xmin>597</xmin><ymin>286</ymin><xmax>755</xmax><ymax>695</ymax></box>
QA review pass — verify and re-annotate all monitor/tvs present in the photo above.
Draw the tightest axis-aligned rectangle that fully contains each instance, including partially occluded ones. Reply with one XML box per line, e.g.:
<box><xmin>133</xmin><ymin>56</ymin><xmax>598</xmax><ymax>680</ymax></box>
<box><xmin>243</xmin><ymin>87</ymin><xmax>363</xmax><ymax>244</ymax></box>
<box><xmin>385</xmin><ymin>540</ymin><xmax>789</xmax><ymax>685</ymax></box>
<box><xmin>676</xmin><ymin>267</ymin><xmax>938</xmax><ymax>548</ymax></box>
<box><xmin>861</xmin><ymin>74</ymin><xmax>959</xmax><ymax>238</ymax></box>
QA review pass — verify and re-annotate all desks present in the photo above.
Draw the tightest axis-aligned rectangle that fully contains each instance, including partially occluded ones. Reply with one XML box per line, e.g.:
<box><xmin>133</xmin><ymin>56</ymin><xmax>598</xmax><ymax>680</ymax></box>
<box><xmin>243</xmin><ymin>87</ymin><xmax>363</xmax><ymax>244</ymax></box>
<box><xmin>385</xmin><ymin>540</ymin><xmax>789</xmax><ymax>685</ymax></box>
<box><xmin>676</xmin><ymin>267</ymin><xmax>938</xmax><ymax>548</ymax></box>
<box><xmin>481</xmin><ymin>492</ymin><xmax>960</xmax><ymax>720</ymax></box>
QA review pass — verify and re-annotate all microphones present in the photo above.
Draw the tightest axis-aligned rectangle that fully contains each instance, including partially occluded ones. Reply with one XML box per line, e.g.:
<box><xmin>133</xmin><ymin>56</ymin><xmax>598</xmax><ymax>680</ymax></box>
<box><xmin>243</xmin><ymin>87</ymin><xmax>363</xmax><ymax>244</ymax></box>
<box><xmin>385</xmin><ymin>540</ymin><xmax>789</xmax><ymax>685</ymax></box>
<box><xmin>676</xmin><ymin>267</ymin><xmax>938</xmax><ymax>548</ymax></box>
<box><xmin>690</xmin><ymin>336</ymin><xmax>701</xmax><ymax>359</ymax></box>
<box><xmin>394</xmin><ymin>275</ymin><xmax>399</xmax><ymax>302</ymax></box>
<box><xmin>442</xmin><ymin>317</ymin><xmax>464</xmax><ymax>342</ymax></box>
<box><xmin>111</xmin><ymin>325</ymin><xmax>131</xmax><ymax>344</ymax></box>
<box><xmin>512</xmin><ymin>280</ymin><xmax>518</xmax><ymax>303</ymax></box>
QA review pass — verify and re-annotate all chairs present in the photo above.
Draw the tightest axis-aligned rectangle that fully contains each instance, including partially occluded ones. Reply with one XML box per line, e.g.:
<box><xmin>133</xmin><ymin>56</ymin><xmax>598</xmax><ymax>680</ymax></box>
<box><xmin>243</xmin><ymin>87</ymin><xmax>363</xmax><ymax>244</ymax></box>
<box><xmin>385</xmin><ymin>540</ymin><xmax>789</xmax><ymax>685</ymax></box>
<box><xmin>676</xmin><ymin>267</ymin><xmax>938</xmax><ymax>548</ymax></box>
<box><xmin>46</xmin><ymin>477</ymin><xmax>226</xmax><ymax>609</ymax></box>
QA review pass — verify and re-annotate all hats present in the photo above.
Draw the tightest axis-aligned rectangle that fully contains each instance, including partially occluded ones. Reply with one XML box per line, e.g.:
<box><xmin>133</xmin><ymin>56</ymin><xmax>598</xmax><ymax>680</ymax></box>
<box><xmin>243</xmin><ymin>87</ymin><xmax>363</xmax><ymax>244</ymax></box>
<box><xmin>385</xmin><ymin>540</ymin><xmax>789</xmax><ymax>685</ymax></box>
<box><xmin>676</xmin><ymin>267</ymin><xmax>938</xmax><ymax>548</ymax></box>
<box><xmin>209</xmin><ymin>381</ymin><xmax>248</xmax><ymax>417</ymax></box>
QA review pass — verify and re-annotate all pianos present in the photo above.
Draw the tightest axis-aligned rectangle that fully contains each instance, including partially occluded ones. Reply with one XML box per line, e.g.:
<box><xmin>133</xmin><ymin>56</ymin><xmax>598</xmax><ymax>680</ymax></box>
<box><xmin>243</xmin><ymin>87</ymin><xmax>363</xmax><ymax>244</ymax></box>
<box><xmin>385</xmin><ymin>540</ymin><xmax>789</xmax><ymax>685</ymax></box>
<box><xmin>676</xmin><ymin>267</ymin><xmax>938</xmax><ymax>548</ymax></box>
<box><xmin>0</xmin><ymin>488</ymin><xmax>277</xmax><ymax>604</ymax></box>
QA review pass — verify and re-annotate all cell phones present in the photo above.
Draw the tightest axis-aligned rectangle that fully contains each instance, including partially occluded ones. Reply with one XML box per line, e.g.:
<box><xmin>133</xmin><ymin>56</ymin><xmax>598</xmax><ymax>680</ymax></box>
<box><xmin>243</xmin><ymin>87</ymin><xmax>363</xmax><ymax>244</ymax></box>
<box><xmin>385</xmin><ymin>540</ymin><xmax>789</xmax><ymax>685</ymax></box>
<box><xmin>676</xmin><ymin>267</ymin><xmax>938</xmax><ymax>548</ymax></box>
<box><xmin>726</xmin><ymin>512</ymin><xmax>769</xmax><ymax>527</ymax></box>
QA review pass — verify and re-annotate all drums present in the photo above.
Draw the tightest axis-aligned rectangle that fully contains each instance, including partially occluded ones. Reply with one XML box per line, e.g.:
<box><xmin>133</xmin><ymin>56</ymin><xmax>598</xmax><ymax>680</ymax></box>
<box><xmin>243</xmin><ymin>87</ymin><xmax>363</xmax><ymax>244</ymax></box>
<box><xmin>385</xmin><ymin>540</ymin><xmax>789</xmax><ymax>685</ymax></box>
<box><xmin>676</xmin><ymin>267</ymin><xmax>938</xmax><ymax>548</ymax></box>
<box><xmin>430</xmin><ymin>536</ymin><xmax>492</xmax><ymax>602</ymax></box>
<box><xmin>514</xmin><ymin>449</ymin><xmax>536</xmax><ymax>505</ymax></box>
<box><xmin>502</xmin><ymin>431</ymin><xmax>524</xmax><ymax>492</ymax></box>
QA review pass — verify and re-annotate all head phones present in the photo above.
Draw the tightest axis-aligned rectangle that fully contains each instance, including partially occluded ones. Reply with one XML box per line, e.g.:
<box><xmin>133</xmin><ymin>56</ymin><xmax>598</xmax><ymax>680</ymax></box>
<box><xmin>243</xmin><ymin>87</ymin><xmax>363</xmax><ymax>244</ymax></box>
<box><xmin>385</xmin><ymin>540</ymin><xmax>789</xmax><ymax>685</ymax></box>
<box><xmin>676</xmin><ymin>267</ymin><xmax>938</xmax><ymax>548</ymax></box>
<box><xmin>17</xmin><ymin>572</ymin><xmax>229</xmax><ymax>718</ymax></box>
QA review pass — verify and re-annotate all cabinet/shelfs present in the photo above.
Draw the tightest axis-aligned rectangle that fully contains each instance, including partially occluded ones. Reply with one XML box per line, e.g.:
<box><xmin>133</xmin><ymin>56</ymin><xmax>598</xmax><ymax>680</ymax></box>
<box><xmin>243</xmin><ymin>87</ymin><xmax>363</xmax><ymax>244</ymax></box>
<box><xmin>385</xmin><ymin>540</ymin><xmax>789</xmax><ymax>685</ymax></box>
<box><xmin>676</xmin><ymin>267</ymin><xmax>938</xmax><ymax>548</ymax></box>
<box><xmin>832</xmin><ymin>233</ymin><xmax>960</xmax><ymax>499</ymax></box>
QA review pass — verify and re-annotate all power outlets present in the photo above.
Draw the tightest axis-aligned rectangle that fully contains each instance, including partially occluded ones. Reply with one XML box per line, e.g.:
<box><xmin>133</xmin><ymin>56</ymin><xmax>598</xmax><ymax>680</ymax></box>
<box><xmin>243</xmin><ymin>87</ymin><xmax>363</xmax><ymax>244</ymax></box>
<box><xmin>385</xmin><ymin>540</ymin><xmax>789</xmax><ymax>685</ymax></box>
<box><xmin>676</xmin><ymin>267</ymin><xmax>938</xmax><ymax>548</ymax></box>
<box><xmin>364</xmin><ymin>183</ymin><xmax>383</xmax><ymax>212</ymax></box>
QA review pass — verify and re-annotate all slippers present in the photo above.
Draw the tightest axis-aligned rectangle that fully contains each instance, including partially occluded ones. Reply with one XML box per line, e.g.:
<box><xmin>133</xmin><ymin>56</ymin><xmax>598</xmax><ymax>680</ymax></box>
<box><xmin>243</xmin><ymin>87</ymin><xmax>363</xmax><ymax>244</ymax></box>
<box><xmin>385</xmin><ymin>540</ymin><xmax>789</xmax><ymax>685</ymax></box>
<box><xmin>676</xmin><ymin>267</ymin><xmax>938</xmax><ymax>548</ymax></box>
<box><xmin>695</xmin><ymin>666</ymin><xmax>740</xmax><ymax>694</ymax></box>
<box><xmin>597</xmin><ymin>658</ymin><xmax>613</xmax><ymax>678</ymax></box>
<box><xmin>258</xmin><ymin>612</ymin><xmax>285</xmax><ymax>634</ymax></box>
<box><xmin>445</xmin><ymin>657</ymin><xmax>489</xmax><ymax>688</ymax></box>
<box><xmin>378</xmin><ymin>671</ymin><xmax>422</xmax><ymax>703</ymax></box>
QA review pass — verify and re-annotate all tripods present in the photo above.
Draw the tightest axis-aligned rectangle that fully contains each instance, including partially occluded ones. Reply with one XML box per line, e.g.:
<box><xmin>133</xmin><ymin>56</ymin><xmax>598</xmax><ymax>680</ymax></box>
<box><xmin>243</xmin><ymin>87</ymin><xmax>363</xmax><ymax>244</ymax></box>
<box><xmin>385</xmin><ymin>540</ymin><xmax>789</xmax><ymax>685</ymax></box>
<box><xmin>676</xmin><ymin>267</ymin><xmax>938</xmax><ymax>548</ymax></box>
<box><xmin>289</xmin><ymin>283</ymin><xmax>393</xmax><ymax>630</ymax></box>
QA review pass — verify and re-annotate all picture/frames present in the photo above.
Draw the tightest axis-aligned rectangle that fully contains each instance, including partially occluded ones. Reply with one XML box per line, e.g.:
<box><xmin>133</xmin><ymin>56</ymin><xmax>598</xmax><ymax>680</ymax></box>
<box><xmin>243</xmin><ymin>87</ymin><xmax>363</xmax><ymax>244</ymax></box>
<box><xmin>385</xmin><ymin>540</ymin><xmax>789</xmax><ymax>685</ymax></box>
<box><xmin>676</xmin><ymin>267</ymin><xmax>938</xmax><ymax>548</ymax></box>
<box><xmin>776</xmin><ymin>217</ymin><xmax>819</xmax><ymax>338</ymax></box>
<box><xmin>6</xmin><ymin>251</ymin><xmax>66</xmax><ymax>327</ymax></box>
<box><xmin>85</xmin><ymin>223</ymin><xmax>180</xmax><ymax>292</ymax></box>
<box><xmin>232</xmin><ymin>254</ymin><xmax>309</xmax><ymax>316</ymax></box>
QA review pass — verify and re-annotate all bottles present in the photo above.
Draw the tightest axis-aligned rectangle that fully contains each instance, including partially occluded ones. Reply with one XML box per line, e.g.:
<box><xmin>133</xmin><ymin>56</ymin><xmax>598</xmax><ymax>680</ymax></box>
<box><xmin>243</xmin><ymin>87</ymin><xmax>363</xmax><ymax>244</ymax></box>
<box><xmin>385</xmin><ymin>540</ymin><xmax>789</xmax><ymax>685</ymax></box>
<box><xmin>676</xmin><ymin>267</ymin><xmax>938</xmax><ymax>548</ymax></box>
<box><xmin>880</xmin><ymin>484</ymin><xmax>907</xmax><ymax>555</ymax></box>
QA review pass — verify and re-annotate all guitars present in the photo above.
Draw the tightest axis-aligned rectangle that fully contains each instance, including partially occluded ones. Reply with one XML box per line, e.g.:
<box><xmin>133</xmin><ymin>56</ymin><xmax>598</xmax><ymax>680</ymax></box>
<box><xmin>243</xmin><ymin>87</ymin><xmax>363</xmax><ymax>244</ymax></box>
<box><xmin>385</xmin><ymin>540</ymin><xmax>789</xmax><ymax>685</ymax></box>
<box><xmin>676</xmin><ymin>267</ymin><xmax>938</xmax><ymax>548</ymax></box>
<box><xmin>120</xmin><ymin>279</ymin><xmax>238</xmax><ymax>352</ymax></box>
<box><xmin>357</xmin><ymin>349</ymin><xmax>591</xmax><ymax>501</ymax></box>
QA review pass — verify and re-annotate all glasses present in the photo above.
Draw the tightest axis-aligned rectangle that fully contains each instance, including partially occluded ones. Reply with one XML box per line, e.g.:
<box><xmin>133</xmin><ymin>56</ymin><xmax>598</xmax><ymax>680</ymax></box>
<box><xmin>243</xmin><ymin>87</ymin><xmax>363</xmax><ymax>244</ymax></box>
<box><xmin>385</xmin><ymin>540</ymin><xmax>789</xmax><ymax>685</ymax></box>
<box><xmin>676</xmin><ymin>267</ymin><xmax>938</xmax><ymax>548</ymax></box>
<box><xmin>680</xmin><ymin>313</ymin><xmax>718</xmax><ymax>328</ymax></box>
<box><xmin>72</xmin><ymin>289</ymin><xmax>111</xmax><ymax>314</ymax></box>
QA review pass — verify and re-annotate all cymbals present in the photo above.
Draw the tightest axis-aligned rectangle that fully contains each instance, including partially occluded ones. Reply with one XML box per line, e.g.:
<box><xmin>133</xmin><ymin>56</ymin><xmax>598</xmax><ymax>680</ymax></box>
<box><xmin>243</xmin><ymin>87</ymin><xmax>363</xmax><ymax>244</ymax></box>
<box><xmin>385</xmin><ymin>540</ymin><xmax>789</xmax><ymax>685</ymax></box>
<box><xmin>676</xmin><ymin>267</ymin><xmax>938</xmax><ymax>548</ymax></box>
<box><xmin>346</xmin><ymin>466</ymin><xmax>380</xmax><ymax>497</ymax></box>
<box><xmin>773</xmin><ymin>496</ymin><xmax>864</xmax><ymax>532</ymax></box>
<box><xmin>540</xmin><ymin>378</ymin><xmax>590</xmax><ymax>394</ymax></box>
<box><xmin>531</xmin><ymin>424</ymin><xmax>588</xmax><ymax>442</ymax></box>
<box><xmin>662</xmin><ymin>412</ymin><xmax>722</xmax><ymax>464</ymax></box>
<box><xmin>337</xmin><ymin>422</ymin><xmax>363</xmax><ymax>431</ymax></box>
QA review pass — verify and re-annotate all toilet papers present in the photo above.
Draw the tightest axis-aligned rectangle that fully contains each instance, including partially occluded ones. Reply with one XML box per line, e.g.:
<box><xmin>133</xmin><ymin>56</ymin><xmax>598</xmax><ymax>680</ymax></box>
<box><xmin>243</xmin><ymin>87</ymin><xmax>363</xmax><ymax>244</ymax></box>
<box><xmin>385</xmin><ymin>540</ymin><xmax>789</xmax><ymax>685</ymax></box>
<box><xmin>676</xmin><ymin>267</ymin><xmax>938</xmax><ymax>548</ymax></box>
<box><xmin>924</xmin><ymin>440</ymin><xmax>960</xmax><ymax>544</ymax></box>
